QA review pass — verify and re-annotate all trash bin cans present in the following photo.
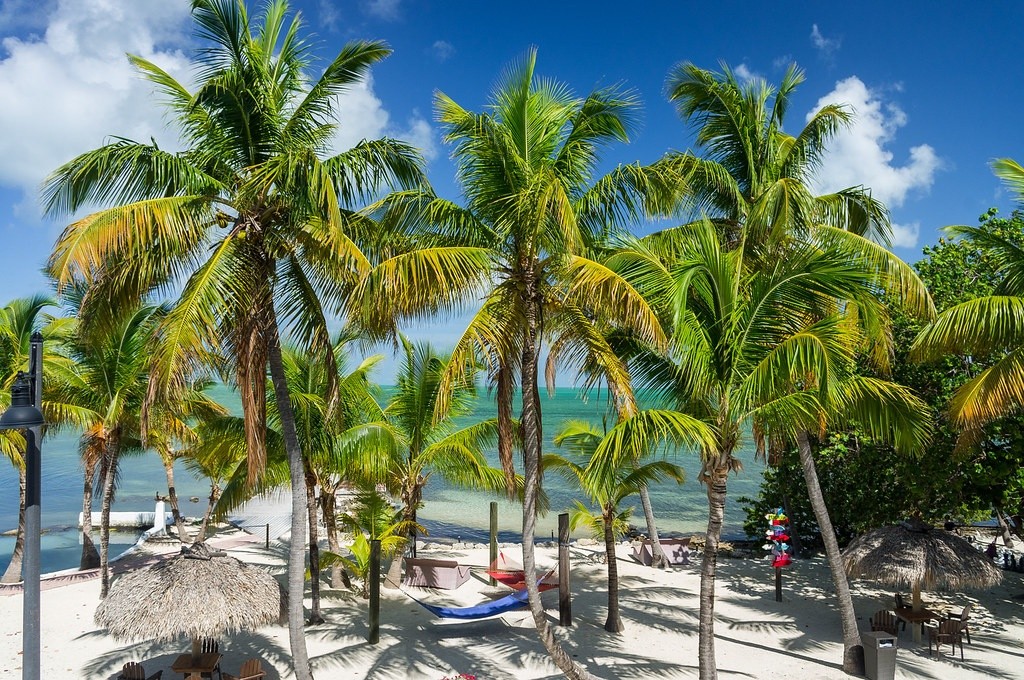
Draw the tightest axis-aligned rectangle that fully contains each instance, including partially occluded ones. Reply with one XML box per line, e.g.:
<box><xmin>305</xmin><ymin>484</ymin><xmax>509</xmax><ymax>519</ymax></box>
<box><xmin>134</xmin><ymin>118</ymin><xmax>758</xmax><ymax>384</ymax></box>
<box><xmin>862</xmin><ymin>631</ymin><xmax>897</xmax><ymax>680</ymax></box>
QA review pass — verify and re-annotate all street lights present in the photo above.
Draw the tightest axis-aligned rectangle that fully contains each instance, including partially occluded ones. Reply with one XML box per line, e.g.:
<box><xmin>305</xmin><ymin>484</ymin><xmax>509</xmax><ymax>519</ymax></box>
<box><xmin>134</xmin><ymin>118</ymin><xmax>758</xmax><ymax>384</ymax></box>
<box><xmin>0</xmin><ymin>332</ymin><xmax>46</xmax><ymax>680</ymax></box>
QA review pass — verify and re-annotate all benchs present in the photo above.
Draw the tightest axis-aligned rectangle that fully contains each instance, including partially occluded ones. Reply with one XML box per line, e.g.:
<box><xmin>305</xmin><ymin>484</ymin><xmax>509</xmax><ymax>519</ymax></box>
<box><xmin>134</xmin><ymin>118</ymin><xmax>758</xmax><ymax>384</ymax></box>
<box><xmin>403</xmin><ymin>555</ymin><xmax>472</xmax><ymax>589</ymax></box>
<box><xmin>629</xmin><ymin>539</ymin><xmax>692</xmax><ymax>566</ymax></box>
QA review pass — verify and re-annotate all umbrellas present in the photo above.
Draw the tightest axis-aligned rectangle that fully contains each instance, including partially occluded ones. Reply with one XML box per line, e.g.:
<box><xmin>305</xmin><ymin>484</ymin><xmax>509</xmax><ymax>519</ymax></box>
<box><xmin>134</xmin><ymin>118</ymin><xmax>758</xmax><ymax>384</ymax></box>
<box><xmin>839</xmin><ymin>507</ymin><xmax>1003</xmax><ymax>642</ymax></box>
<box><xmin>93</xmin><ymin>541</ymin><xmax>289</xmax><ymax>680</ymax></box>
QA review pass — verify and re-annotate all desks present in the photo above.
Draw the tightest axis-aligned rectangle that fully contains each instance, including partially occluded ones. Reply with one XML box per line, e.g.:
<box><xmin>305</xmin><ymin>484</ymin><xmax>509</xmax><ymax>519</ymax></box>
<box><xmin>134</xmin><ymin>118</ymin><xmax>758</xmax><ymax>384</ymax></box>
<box><xmin>893</xmin><ymin>607</ymin><xmax>940</xmax><ymax>624</ymax></box>
<box><xmin>171</xmin><ymin>653</ymin><xmax>221</xmax><ymax>680</ymax></box>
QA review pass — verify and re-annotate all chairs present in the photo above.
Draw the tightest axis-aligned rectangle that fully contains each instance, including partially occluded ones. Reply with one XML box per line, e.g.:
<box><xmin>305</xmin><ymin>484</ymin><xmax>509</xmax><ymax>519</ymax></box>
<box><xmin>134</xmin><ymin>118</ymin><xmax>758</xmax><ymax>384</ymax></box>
<box><xmin>183</xmin><ymin>636</ymin><xmax>222</xmax><ymax>680</ymax></box>
<box><xmin>869</xmin><ymin>609</ymin><xmax>898</xmax><ymax>637</ymax></box>
<box><xmin>895</xmin><ymin>593</ymin><xmax>925</xmax><ymax>635</ymax></box>
<box><xmin>927</xmin><ymin>619</ymin><xmax>965</xmax><ymax>663</ymax></box>
<box><xmin>116</xmin><ymin>661</ymin><xmax>164</xmax><ymax>680</ymax></box>
<box><xmin>222</xmin><ymin>657</ymin><xmax>266</xmax><ymax>680</ymax></box>
<box><xmin>938</xmin><ymin>605</ymin><xmax>972</xmax><ymax>645</ymax></box>
